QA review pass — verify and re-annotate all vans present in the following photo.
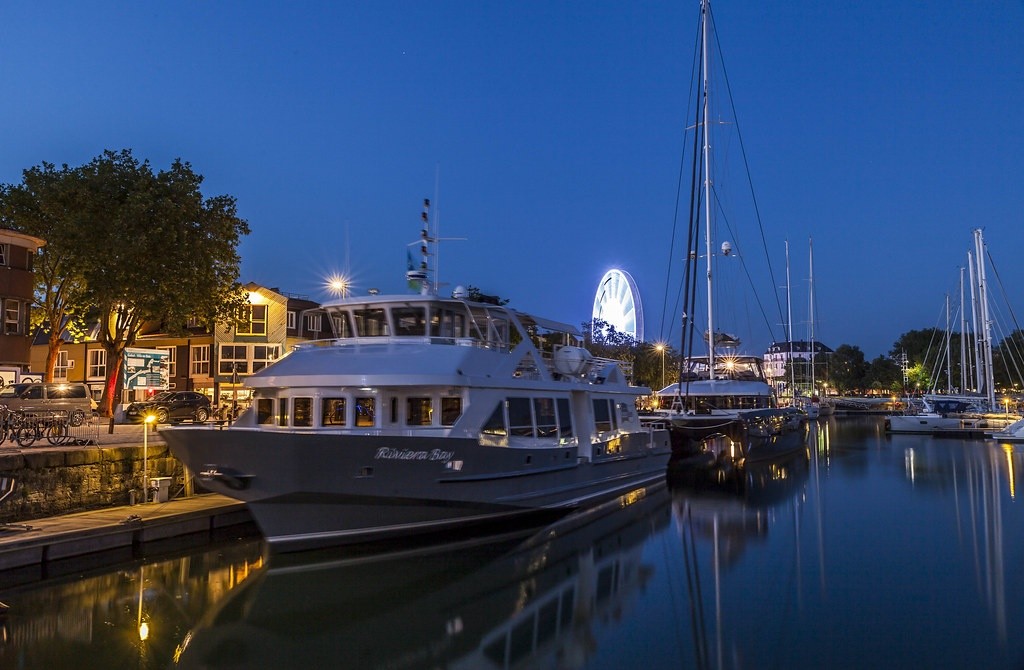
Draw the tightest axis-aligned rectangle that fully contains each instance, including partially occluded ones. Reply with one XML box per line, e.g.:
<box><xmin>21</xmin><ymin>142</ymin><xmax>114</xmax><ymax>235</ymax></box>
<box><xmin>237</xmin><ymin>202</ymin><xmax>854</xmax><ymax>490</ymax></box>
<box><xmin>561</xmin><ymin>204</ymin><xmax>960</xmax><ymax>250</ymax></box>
<box><xmin>0</xmin><ymin>383</ymin><xmax>93</xmax><ymax>427</ymax></box>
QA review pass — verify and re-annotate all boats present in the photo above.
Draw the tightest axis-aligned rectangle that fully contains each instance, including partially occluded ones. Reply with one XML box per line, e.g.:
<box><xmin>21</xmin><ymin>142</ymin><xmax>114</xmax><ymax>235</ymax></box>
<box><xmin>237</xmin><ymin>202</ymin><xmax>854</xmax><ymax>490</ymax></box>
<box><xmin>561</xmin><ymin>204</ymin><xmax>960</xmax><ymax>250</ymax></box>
<box><xmin>156</xmin><ymin>158</ymin><xmax>670</xmax><ymax>551</ymax></box>
<box><xmin>176</xmin><ymin>482</ymin><xmax>673</xmax><ymax>670</ymax></box>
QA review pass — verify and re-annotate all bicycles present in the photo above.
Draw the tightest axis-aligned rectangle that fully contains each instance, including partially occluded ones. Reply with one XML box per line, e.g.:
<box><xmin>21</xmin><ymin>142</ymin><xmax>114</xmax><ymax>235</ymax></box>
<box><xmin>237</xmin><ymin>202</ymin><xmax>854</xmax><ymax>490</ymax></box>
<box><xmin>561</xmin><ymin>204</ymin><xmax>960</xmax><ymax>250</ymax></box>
<box><xmin>0</xmin><ymin>405</ymin><xmax>67</xmax><ymax>448</ymax></box>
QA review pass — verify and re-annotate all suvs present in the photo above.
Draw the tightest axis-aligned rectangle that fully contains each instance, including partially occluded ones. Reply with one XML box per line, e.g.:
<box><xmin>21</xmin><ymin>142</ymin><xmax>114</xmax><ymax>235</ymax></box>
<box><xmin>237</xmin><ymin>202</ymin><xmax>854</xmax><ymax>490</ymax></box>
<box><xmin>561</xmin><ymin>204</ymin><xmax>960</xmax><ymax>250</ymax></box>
<box><xmin>127</xmin><ymin>391</ymin><xmax>211</xmax><ymax>424</ymax></box>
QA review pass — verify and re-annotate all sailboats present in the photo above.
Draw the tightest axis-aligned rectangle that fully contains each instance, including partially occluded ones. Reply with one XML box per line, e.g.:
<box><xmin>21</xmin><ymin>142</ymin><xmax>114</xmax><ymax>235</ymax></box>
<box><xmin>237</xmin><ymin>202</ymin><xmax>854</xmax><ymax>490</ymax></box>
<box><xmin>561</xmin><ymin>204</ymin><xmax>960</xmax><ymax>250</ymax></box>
<box><xmin>638</xmin><ymin>0</ymin><xmax>835</xmax><ymax>441</ymax></box>
<box><xmin>884</xmin><ymin>226</ymin><xmax>1024</xmax><ymax>440</ymax></box>
